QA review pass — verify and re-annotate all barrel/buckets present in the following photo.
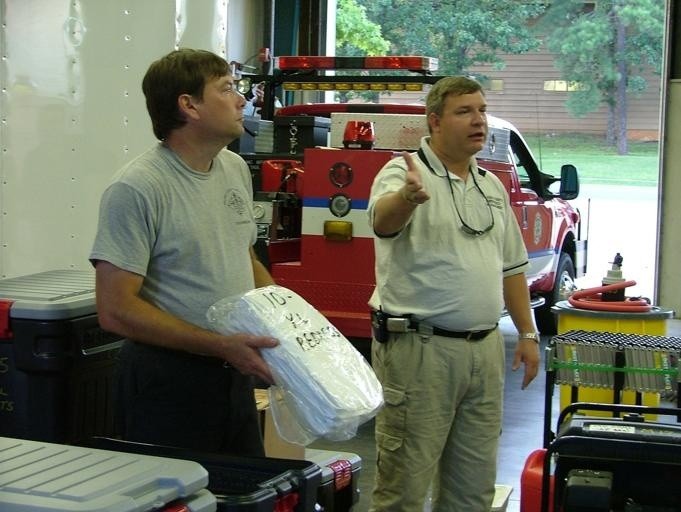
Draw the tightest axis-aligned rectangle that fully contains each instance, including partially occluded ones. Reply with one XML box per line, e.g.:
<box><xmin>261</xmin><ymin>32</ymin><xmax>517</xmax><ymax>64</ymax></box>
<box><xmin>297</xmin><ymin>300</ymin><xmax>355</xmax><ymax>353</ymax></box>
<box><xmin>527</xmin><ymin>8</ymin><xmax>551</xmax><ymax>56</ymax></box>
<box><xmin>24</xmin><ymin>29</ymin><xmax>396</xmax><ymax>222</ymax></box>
<box><xmin>550</xmin><ymin>301</ymin><xmax>674</xmax><ymax>422</ymax></box>
<box><xmin>520</xmin><ymin>447</ymin><xmax>555</xmax><ymax>512</ymax></box>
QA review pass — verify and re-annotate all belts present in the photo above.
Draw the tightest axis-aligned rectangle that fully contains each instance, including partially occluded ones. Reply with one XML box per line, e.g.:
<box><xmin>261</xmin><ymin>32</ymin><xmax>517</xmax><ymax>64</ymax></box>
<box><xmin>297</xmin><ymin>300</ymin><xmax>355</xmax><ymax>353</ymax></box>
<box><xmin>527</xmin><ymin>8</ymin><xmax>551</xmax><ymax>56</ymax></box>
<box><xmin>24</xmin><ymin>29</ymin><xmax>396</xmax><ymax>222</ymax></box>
<box><xmin>409</xmin><ymin>318</ymin><xmax>500</xmax><ymax>342</ymax></box>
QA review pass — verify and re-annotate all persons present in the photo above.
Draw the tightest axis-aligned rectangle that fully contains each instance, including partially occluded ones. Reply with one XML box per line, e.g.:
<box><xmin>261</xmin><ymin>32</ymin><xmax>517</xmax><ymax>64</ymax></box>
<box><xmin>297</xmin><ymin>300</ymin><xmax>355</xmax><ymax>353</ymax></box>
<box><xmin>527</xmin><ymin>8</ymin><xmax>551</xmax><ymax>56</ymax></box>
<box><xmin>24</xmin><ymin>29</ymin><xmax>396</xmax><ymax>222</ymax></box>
<box><xmin>88</xmin><ymin>46</ymin><xmax>276</xmax><ymax>461</ymax></box>
<box><xmin>368</xmin><ymin>76</ymin><xmax>540</xmax><ymax>512</ymax></box>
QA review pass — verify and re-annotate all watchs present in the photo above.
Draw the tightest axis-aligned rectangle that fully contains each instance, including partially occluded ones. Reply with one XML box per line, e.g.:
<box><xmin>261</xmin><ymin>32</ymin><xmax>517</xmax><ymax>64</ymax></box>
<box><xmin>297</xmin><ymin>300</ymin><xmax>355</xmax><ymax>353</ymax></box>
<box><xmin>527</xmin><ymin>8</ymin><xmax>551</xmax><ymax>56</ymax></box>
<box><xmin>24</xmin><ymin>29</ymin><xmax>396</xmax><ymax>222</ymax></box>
<box><xmin>516</xmin><ymin>332</ymin><xmax>541</xmax><ymax>344</ymax></box>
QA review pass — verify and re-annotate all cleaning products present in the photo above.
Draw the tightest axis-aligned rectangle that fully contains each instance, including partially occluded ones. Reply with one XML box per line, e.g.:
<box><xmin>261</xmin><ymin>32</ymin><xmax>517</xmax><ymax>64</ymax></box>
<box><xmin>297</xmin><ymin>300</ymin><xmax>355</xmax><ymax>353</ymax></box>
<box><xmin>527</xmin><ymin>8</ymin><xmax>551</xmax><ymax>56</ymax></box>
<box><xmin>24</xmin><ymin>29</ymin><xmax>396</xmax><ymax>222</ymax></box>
<box><xmin>602</xmin><ymin>253</ymin><xmax>626</xmax><ymax>303</ymax></box>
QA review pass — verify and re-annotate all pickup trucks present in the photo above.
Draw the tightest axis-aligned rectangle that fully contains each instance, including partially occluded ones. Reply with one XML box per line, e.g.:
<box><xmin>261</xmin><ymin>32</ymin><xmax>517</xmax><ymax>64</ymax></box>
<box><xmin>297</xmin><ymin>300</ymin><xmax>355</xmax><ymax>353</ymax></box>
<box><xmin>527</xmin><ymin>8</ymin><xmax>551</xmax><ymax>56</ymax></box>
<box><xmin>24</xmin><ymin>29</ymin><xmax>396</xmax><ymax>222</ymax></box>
<box><xmin>236</xmin><ymin>55</ymin><xmax>591</xmax><ymax>340</ymax></box>
